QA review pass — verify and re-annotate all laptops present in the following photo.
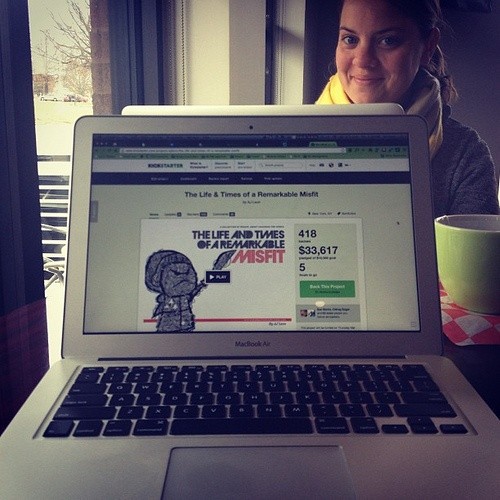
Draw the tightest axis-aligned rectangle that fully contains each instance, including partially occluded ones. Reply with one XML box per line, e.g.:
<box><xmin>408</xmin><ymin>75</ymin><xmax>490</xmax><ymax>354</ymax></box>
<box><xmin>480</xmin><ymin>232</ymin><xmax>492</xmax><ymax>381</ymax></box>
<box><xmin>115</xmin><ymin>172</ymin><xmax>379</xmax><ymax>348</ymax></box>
<box><xmin>0</xmin><ymin>102</ymin><xmax>500</xmax><ymax>500</ymax></box>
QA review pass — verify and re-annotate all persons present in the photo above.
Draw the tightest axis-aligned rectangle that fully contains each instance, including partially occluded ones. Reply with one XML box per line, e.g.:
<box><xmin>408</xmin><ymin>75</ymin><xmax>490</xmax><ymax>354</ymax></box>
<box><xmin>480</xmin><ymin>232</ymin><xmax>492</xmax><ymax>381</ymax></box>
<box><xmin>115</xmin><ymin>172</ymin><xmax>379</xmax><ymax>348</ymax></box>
<box><xmin>314</xmin><ymin>0</ymin><xmax>500</xmax><ymax>216</ymax></box>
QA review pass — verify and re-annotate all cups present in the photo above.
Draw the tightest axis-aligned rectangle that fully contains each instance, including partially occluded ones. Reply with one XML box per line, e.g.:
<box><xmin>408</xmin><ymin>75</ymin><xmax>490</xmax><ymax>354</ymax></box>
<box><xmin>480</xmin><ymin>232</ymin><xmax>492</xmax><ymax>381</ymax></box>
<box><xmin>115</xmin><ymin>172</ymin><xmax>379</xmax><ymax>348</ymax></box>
<box><xmin>433</xmin><ymin>214</ymin><xmax>500</xmax><ymax>317</ymax></box>
